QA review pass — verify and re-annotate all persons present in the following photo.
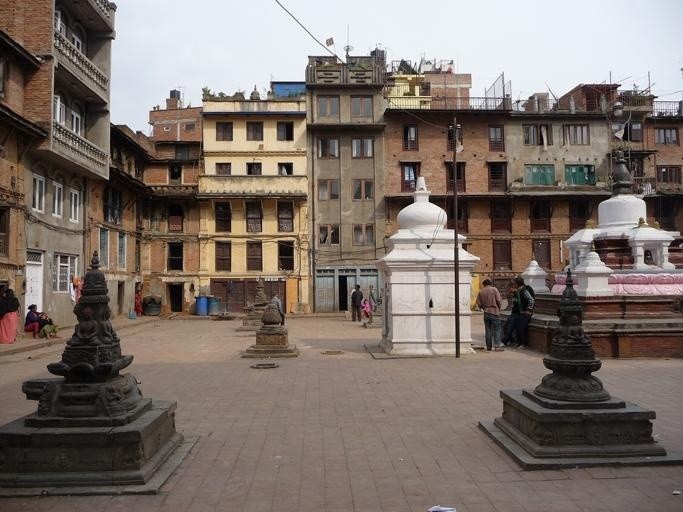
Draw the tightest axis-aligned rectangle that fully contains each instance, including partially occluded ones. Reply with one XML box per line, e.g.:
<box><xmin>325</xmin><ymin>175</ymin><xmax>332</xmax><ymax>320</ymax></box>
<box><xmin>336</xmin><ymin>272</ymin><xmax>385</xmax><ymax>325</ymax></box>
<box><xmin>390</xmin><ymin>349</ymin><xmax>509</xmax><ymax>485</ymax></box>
<box><xmin>135</xmin><ymin>290</ymin><xmax>143</xmax><ymax>317</ymax></box>
<box><xmin>24</xmin><ymin>304</ymin><xmax>48</xmax><ymax>339</ymax></box>
<box><xmin>271</xmin><ymin>293</ymin><xmax>284</xmax><ymax>326</ymax></box>
<box><xmin>475</xmin><ymin>279</ymin><xmax>504</xmax><ymax>352</ymax></box>
<box><xmin>0</xmin><ymin>289</ymin><xmax>22</xmax><ymax>344</ymax></box>
<box><xmin>493</xmin><ymin>283</ymin><xmax>518</xmax><ymax>348</ymax></box>
<box><xmin>38</xmin><ymin>312</ymin><xmax>62</xmax><ymax>340</ymax></box>
<box><xmin>512</xmin><ymin>277</ymin><xmax>534</xmax><ymax>350</ymax></box>
<box><xmin>350</xmin><ymin>284</ymin><xmax>377</xmax><ymax>322</ymax></box>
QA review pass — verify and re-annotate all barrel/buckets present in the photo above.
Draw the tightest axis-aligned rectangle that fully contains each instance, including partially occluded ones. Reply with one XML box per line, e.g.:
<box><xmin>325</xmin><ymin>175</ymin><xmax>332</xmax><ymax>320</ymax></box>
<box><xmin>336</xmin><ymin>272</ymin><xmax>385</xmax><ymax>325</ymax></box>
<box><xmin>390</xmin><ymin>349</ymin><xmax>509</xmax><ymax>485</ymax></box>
<box><xmin>194</xmin><ymin>296</ymin><xmax>208</xmax><ymax>316</ymax></box>
<box><xmin>207</xmin><ymin>297</ymin><xmax>221</xmax><ymax>316</ymax></box>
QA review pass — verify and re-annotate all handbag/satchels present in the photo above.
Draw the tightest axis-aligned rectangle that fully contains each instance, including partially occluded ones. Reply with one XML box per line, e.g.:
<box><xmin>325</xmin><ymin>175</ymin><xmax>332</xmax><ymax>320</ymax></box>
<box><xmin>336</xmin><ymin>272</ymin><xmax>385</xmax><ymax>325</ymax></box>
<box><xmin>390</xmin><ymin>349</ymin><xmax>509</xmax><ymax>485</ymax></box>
<box><xmin>521</xmin><ymin>286</ymin><xmax>536</xmax><ymax>306</ymax></box>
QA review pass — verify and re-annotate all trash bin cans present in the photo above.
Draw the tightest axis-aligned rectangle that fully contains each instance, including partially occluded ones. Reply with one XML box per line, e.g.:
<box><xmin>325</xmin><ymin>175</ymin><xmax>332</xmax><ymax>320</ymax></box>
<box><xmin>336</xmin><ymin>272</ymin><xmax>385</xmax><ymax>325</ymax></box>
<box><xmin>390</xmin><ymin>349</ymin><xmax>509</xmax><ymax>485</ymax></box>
<box><xmin>143</xmin><ymin>295</ymin><xmax>161</xmax><ymax>316</ymax></box>
<box><xmin>195</xmin><ymin>295</ymin><xmax>222</xmax><ymax>316</ymax></box>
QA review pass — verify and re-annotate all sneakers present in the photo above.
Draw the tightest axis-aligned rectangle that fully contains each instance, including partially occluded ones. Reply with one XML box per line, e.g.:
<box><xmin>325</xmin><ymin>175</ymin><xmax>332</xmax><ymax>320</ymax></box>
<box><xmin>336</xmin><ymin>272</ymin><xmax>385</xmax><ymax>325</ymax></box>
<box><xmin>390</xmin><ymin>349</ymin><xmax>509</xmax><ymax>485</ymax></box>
<box><xmin>487</xmin><ymin>341</ymin><xmax>528</xmax><ymax>351</ymax></box>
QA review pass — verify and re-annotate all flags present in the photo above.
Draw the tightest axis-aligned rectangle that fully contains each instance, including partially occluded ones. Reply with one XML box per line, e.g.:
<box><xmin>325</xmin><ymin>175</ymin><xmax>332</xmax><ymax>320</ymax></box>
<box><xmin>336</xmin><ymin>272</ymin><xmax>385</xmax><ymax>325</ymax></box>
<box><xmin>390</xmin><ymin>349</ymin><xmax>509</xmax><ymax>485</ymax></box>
<box><xmin>325</xmin><ymin>37</ymin><xmax>335</xmax><ymax>47</ymax></box>
